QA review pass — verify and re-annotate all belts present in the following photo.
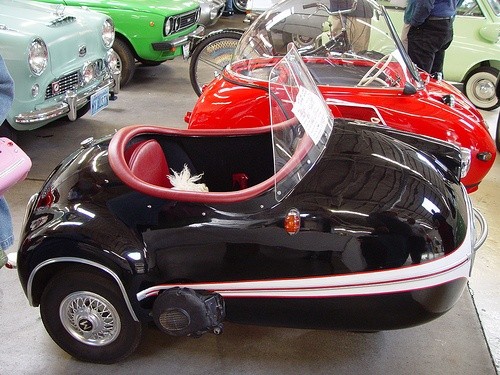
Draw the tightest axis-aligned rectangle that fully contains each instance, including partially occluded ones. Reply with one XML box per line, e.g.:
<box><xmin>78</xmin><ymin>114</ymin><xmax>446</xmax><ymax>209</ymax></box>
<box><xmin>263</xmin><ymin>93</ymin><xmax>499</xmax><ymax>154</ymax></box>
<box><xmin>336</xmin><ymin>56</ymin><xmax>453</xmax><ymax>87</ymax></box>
<box><xmin>427</xmin><ymin>16</ymin><xmax>455</xmax><ymax>20</ymax></box>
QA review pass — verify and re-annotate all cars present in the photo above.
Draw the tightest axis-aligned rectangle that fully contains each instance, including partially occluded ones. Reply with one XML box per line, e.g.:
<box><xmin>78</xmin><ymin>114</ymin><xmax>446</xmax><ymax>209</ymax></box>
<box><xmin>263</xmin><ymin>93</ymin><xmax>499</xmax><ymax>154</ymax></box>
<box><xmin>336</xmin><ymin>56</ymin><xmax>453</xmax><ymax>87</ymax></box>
<box><xmin>16</xmin><ymin>42</ymin><xmax>489</xmax><ymax>365</ymax></box>
<box><xmin>0</xmin><ymin>0</ymin><xmax>500</xmax><ymax>197</ymax></box>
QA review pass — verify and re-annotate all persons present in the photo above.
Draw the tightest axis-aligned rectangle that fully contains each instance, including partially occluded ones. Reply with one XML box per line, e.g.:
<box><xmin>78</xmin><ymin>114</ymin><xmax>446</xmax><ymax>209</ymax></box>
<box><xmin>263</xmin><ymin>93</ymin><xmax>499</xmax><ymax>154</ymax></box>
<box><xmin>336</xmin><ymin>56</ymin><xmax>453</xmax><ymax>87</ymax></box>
<box><xmin>0</xmin><ymin>54</ymin><xmax>14</xmax><ymax>272</ymax></box>
<box><xmin>330</xmin><ymin>0</ymin><xmax>373</xmax><ymax>54</ymax></box>
<box><xmin>401</xmin><ymin>0</ymin><xmax>464</xmax><ymax>79</ymax></box>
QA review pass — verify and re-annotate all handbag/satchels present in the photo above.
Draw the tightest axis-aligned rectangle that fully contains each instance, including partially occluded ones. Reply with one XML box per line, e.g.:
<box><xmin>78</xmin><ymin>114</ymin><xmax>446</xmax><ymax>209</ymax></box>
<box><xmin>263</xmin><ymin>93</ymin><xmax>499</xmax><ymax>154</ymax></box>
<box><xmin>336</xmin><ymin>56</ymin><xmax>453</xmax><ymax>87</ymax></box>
<box><xmin>0</xmin><ymin>137</ymin><xmax>32</xmax><ymax>196</ymax></box>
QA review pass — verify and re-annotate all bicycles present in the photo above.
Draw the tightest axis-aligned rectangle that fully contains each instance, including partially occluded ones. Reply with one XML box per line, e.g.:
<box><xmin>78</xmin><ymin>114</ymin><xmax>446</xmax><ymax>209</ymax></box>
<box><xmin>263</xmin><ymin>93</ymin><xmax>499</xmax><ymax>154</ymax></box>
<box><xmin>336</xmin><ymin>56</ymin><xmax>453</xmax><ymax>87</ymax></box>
<box><xmin>185</xmin><ymin>0</ymin><xmax>358</xmax><ymax>98</ymax></box>
